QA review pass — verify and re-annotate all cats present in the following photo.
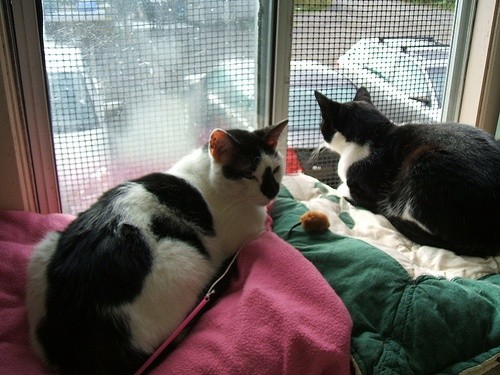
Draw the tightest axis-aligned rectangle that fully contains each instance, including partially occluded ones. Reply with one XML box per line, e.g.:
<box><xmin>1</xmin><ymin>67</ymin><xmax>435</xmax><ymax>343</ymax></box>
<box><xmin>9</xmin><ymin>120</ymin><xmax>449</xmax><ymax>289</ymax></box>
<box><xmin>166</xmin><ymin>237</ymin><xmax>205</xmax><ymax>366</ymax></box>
<box><xmin>27</xmin><ymin>118</ymin><xmax>288</xmax><ymax>375</ymax></box>
<box><xmin>314</xmin><ymin>85</ymin><xmax>500</xmax><ymax>258</ymax></box>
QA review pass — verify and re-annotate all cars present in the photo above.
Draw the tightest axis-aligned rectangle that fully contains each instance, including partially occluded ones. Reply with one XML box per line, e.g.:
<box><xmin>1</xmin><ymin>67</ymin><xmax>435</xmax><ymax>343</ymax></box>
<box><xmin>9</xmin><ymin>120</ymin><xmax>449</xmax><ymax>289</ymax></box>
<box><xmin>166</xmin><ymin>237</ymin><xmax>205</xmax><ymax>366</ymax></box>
<box><xmin>41</xmin><ymin>40</ymin><xmax>122</xmax><ymax>217</ymax></box>
<box><xmin>186</xmin><ymin>59</ymin><xmax>378</xmax><ymax>186</ymax></box>
<box><xmin>334</xmin><ymin>34</ymin><xmax>451</xmax><ymax>124</ymax></box>
